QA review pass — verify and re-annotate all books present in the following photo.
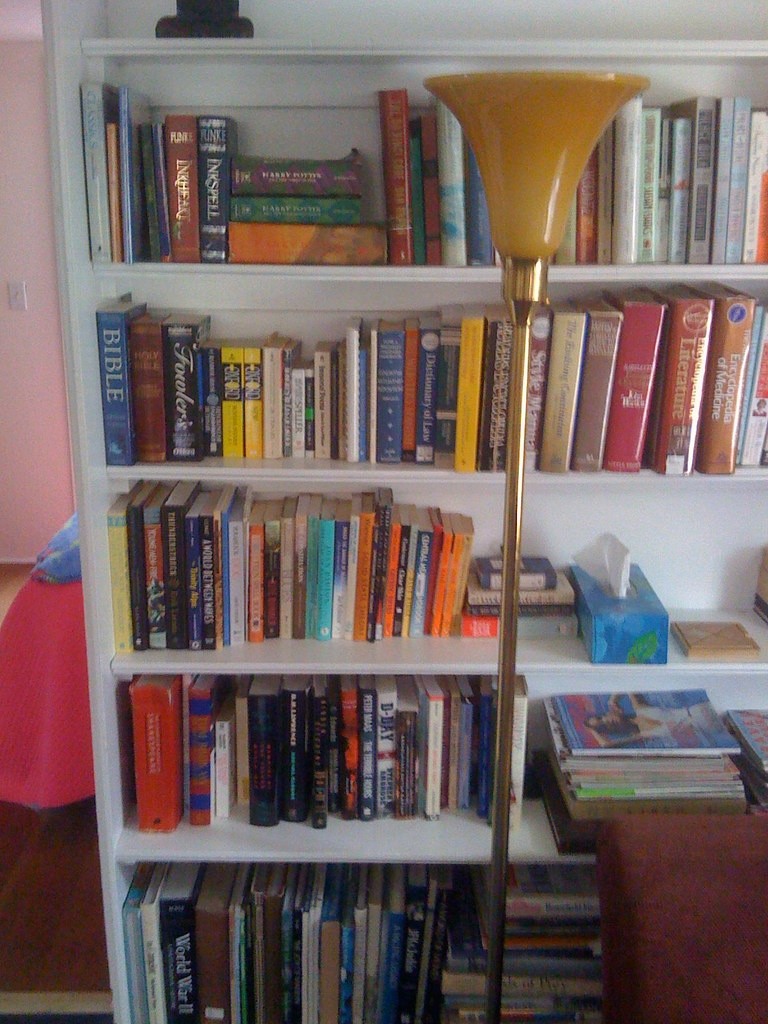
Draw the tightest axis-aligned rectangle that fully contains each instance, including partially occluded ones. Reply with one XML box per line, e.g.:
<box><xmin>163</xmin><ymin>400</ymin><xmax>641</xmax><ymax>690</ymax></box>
<box><xmin>121</xmin><ymin>863</ymin><xmax>603</xmax><ymax>1024</ymax></box>
<box><xmin>95</xmin><ymin>280</ymin><xmax>768</xmax><ymax>474</ymax></box>
<box><xmin>129</xmin><ymin>675</ymin><xmax>529</xmax><ymax>833</ymax></box>
<box><xmin>81</xmin><ymin>81</ymin><xmax>768</xmax><ymax>266</ymax></box>
<box><xmin>537</xmin><ymin>688</ymin><xmax>768</xmax><ymax>856</ymax></box>
<box><xmin>106</xmin><ymin>480</ymin><xmax>579</xmax><ymax>659</ymax></box>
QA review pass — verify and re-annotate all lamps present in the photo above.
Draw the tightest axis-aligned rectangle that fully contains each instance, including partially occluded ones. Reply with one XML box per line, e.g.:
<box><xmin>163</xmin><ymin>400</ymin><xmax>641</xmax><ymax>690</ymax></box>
<box><xmin>423</xmin><ymin>70</ymin><xmax>650</xmax><ymax>1024</ymax></box>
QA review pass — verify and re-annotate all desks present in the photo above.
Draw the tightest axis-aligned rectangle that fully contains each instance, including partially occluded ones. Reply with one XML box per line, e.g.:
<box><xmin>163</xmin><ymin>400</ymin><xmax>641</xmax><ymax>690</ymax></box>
<box><xmin>601</xmin><ymin>815</ymin><xmax>768</xmax><ymax>1024</ymax></box>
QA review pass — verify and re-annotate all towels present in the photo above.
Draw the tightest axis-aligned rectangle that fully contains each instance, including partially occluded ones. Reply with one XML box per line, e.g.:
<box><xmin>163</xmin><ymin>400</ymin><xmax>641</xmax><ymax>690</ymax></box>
<box><xmin>28</xmin><ymin>509</ymin><xmax>81</xmax><ymax>587</ymax></box>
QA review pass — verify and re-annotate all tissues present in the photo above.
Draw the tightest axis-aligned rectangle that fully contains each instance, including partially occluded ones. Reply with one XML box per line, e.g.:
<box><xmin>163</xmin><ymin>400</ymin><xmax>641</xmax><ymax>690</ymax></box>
<box><xmin>568</xmin><ymin>530</ymin><xmax>670</xmax><ymax>665</ymax></box>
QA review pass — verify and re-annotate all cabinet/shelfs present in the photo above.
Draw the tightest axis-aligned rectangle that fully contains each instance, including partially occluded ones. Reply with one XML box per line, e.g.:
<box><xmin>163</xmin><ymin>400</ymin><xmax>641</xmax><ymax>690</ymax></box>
<box><xmin>42</xmin><ymin>0</ymin><xmax>768</xmax><ymax>1024</ymax></box>
